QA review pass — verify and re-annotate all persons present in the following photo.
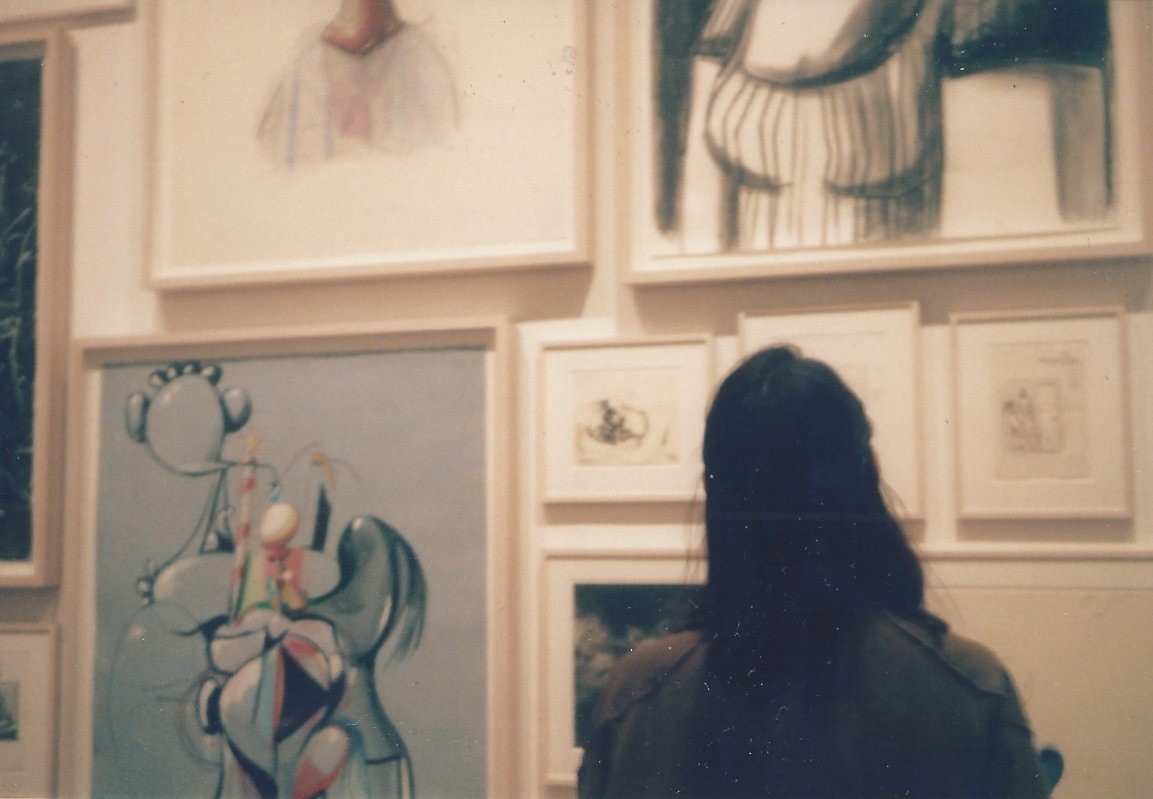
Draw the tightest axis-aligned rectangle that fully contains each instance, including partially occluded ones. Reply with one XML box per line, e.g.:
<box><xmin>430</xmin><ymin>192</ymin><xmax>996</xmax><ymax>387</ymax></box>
<box><xmin>573</xmin><ymin>341</ymin><xmax>1051</xmax><ymax>799</ymax></box>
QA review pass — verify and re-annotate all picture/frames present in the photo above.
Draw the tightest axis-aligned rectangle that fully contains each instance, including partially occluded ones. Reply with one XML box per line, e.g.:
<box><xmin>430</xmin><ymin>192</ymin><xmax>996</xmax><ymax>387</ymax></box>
<box><xmin>0</xmin><ymin>620</ymin><xmax>60</xmax><ymax>797</ymax></box>
<box><xmin>735</xmin><ymin>295</ymin><xmax>932</xmax><ymax>521</ymax></box>
<box><xmin>902</xmin><ymin>542</ymin><xmax>1153</xmax><ymax>799</ymax></box>
<box><xmin>611</xmin><ymin>0</ymin><xmax>1153</xmax><ymax>282</ymax></box>
<box><xmin>1</xmin><ymin>0</ymin><xmax>138</xmax><ymax>30</ymax></box>
<box><xmin>56</xmin><ymin>311</ymin><xmax>522</xmax><ymax>797</ymax></box>
<box><xmin>534</xmin><ymin>544</ymin><xmax>710</xmax><ymax>785</ymax></box>
<box><xmin>950</xmin><ymin>304</ymin><xmax>1139</xmax><ymax>525</ymax></box>
<box><xmin>531</xmin><ymin>328</ymin><xmax>723</xmax><ymax>503</ymax></box>
<box><xmin>143</xmin><ymin>0</ymin><xmax>593</xmax><ymax>298</ymax></box>
<box><xmin>0</xmin><ymin>24</ymin><xmax>73</xmax><ymax>591</ymax></box>
